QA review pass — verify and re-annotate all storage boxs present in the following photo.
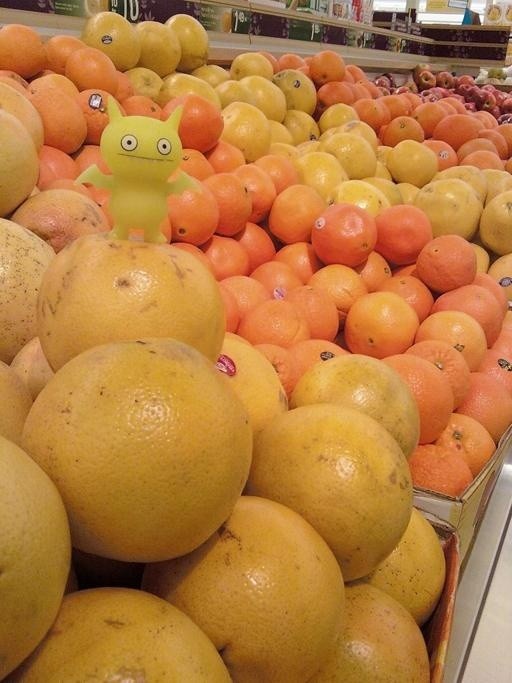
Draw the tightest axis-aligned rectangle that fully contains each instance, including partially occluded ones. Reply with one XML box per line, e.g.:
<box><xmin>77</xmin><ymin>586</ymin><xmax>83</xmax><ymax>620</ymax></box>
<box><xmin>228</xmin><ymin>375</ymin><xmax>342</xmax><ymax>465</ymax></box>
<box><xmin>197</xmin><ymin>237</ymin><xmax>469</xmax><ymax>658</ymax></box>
<box><xmin>412</xmin><ymin>422</ymin><xmax>512</xmax><ymax>683</ymax></box>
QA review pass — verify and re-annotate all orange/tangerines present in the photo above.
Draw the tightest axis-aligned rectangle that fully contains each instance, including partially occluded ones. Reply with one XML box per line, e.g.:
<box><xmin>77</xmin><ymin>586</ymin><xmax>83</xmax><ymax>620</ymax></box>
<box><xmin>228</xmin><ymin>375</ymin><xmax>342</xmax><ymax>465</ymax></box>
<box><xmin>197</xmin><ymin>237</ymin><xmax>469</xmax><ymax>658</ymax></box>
<box><xmin>1</xmin><ymin>26</ymin><xmax>512</xmax><ymax>495</ymax></box>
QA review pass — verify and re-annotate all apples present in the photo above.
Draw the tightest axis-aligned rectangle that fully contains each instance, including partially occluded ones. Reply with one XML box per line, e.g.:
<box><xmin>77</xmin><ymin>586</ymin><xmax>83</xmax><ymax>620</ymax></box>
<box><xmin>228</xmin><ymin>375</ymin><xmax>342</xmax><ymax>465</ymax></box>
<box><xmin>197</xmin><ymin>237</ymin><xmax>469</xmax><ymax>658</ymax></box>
<box><xmin>374</xmin><ymin>62</ymin><xmax>512</xmax><ymax>125</ymax></box>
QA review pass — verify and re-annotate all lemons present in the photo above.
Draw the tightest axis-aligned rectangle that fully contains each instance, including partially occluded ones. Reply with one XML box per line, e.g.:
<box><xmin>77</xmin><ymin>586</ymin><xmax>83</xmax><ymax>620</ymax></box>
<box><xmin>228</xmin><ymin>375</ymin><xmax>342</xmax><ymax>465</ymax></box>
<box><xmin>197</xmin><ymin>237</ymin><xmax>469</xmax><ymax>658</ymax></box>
<box><xmin>83</xmin><ymin>11</ymin><xmax>512</xmax><ymax>299</ymax></box>
<box><xmin>0</xmin><ymin>83</ymin><xmax>446</xmax><ymax>681</ymax></box>
